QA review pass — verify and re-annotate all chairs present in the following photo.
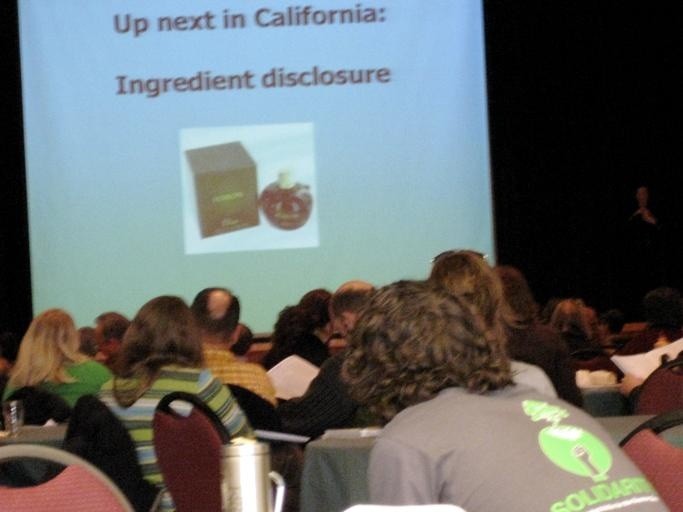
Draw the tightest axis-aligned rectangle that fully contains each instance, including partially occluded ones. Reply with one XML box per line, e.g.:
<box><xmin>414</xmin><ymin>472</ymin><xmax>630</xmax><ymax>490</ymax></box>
<box><xmin>617</xmin><ymin>361</ymin><xmax>681</xmax><ymax>512</ymax></box>
<box><xmin>0</xmin><ymin>392</ymin><xmax>231</xmax><ymax>512</ymax></box>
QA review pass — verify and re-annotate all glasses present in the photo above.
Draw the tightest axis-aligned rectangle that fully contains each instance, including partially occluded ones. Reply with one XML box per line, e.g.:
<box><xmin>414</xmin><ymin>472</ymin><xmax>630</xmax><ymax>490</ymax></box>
<box><xmin>431</xmin><ymin>249</ymin><xmax>484</xmax><ymax>263</ymax></box>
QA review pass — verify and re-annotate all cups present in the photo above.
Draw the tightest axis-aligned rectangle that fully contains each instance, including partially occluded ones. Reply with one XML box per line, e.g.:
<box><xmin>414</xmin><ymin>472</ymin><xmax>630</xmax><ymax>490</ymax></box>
<box><xmin>217</xmin><ymin>440</ymin><xmax>286</xmax><ymax>511</ymax></box>
<box><xmin>2</xmin><ymin>400</ymin><xmax>25</xmax><ymax>440</ymax></box>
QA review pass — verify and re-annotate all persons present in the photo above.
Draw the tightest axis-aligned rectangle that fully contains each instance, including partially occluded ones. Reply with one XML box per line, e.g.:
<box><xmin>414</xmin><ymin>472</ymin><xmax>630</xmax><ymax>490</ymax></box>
<box><xmin>2</xmin><ymin>249</ymin><xmax>683</xmax><ymax>512</ymax></box>
<box><xmin>617</xmin><ymin>181</ymin><xmax>659</xmax><ymax>316</ymax></box>
